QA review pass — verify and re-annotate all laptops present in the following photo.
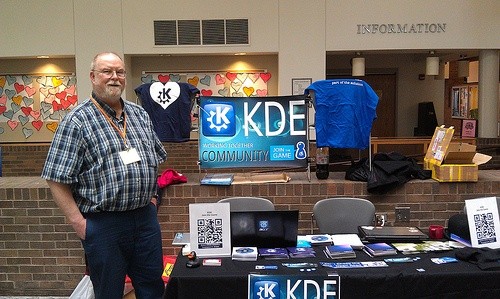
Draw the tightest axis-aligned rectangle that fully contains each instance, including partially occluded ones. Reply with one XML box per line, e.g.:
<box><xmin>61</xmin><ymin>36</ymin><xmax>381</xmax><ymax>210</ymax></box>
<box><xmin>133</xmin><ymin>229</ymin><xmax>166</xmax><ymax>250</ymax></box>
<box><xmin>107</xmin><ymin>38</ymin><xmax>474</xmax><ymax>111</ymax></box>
<box><xmin>358</xmin><ymin>225</ymin><xmax>430</xmax><ymax>243</ymax></box>
<box><xmin>230</xmin><ymin>210</ymin><xmax>299</xmax><ymax>249</ymax></box>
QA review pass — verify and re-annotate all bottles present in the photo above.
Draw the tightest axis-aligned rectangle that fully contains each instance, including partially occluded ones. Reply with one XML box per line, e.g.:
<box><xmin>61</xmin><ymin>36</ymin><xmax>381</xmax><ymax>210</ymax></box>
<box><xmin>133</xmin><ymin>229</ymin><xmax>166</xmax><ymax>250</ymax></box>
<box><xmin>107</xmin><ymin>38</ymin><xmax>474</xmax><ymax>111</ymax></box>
<box><xmin>316</xmin><ymin>147</ymin><xmax>329</xmax><ymax>179</ymax></box>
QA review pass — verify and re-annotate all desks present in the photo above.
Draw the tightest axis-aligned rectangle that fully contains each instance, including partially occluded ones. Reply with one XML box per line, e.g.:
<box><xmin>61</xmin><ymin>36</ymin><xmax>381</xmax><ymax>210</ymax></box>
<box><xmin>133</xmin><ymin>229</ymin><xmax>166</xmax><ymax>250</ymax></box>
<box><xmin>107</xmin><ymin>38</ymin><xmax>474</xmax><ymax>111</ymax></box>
<box><xmin>168</xmin><ymin>224</ymin><xmax>500</xmax><ymax>298</ymax></box>
<box><xmin>371</xmin><ymin>135</ymin><xmax>477</xmax><ymax>156</ymax></box>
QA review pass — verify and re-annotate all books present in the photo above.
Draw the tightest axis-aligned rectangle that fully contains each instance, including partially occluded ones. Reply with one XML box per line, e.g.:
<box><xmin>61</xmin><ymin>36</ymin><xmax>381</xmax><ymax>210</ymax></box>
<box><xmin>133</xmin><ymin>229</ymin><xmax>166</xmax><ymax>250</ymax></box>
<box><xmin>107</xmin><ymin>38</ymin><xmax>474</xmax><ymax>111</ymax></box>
<box><xmin>361</xmin><ymin>243</ymin><xmax>398</xmax><ymax>258</ymax></box>
<box><xmin>203</xmin><ymin>259</ymin><xmax>222</xmax><ymax>266</ymax></box>
<box><xmin>460</xmin><ymin>87</ymin><xmax>472</xmax><ymax>117</ymax></box>
<box><xmin>309</xmin><ymin>233</ymin><xmax>363</xmax><ymax>249</ymax></box>
<box><xmin>201</xmin><ymin>173</ymin><xmax>234</xmax><ymax>185</ymax></box>
<box><xmin>232</xmin><ymin>235</ymin><xmax>316</xmax><ymax>261</ymax></box>
<box><xmin>452</xmin><ymin>88</ymin><xmax>459</xmax><ymax>116</ymax></box>
<box><xmin>393</xmin><ymin>233</ymin><xmax>472</xmax><ymax>255</ymax></box>
<box><xmin>182</xmin><ymin>244</ymin><xmax>190</xmax><ymax>256</ymax></box>
<box><xmin>323</xmin><ymin>245</ymin><xmax>356</xmax><ymax>259</ymax></box>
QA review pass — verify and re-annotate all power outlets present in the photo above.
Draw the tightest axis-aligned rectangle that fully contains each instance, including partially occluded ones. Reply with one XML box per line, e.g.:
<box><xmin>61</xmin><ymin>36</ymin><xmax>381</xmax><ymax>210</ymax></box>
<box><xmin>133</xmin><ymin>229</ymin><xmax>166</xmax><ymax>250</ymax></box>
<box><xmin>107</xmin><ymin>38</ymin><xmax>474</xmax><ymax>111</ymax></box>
<box><xmin>394</xmin><ymin>206</ymin><xmax>411</xmax><ymax>222</ymax></box>
<box><xmin>375</xmin><ymin>212</ymin><xmax>387</xmax><ymax>224</ymax></box>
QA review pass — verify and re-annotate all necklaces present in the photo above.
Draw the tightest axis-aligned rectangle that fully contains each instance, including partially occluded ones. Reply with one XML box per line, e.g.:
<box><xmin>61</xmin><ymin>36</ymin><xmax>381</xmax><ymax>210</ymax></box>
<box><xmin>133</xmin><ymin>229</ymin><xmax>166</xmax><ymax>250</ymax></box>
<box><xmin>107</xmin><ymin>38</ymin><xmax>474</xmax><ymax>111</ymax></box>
<box><xmin>89</xmin><ymin>93</ymin><xmax>142</xmax><ymax>165</ymax></box>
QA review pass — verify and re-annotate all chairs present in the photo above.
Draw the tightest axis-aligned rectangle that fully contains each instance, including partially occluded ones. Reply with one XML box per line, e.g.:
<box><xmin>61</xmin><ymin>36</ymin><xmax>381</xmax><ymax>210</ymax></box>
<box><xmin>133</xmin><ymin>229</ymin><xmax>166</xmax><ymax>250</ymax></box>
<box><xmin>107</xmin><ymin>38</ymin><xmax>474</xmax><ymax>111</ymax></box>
<box><xmin>312</xmin><ymin>198</ymin><xmax>376</xmax><ymax>234</ymax></box>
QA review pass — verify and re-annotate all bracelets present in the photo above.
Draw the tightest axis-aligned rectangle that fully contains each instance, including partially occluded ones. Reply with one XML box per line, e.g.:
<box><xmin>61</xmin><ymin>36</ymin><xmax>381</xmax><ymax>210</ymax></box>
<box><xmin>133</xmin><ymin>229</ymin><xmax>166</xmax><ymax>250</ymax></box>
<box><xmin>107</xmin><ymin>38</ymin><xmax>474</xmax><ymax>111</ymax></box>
<box><xmin>153</xmin><ymin>193</ymin><xmax>159</xmax><ymax>201</ymax></box>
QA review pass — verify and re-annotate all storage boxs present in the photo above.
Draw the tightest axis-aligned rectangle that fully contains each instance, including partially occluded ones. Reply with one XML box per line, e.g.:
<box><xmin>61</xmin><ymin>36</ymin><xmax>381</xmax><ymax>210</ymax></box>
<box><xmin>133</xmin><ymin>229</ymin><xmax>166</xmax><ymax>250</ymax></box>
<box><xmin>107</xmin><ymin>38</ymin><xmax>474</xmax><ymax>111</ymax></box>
<box><xmin>423</xmin><ymin>125</ymin><xmax>493</xmax><ymax>182</ymax></box>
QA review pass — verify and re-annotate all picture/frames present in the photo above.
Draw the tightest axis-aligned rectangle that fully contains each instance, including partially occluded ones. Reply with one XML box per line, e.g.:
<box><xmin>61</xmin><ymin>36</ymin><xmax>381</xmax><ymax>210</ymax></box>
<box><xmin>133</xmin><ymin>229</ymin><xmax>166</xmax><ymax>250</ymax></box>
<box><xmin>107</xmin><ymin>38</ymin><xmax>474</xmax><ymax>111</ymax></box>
<box><xmin>291</xmin><ymin>78</ymin><xmax>312</xmax><ymax>96</ymax></box>
<box><xmin>460</xmin><ymin>119</ymin><xmax>478</xmax><ymax>137</ymax></box>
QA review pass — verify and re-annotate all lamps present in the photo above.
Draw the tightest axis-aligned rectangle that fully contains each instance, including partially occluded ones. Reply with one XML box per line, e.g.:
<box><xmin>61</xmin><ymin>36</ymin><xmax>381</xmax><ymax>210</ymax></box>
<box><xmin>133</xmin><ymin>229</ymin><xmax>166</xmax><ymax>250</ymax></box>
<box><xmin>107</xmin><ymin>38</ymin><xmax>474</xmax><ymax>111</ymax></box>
<box><xmin>350</xmin><ymin>51</ymin><xmax>366</xmax><ymax>77</ymax></box>
<box><xmin>424</xmin><ymin>50</ymin><xmax>440</xmax><ymax>76</ymax></box>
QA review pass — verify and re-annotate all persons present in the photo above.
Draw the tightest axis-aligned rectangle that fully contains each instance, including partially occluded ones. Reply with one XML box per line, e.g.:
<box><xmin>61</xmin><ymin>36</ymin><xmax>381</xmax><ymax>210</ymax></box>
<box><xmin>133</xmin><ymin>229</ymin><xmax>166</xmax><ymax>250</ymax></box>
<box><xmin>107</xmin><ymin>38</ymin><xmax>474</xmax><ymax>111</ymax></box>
<box><xmin>40</xmin><ymin>52</ymin><xmax>168</xmax><ymax>299</ymax></box>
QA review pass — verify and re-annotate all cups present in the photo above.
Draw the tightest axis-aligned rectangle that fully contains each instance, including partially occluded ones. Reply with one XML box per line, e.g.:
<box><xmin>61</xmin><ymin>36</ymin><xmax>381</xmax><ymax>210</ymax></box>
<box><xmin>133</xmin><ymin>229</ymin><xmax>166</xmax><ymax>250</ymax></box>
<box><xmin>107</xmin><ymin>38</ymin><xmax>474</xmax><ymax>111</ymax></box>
<box><xmin>428</xmin><ymin>225</ymin><xmax>444</xmax><ymax>238</ymax></box>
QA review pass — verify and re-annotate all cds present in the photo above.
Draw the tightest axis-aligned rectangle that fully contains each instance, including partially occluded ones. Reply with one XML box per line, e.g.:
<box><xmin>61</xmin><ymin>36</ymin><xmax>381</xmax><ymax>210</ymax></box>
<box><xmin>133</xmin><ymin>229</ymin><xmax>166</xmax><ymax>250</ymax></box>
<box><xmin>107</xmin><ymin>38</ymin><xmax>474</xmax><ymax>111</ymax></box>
<box><xmin>311</xmin><ymin>236</ymin><xmax>328</xmax><ymax>241</ymax></box>
<box><xmin>236</xmin><ymin>248</ymin><xmax>254</xmax><ymax>254</ymax></box>
